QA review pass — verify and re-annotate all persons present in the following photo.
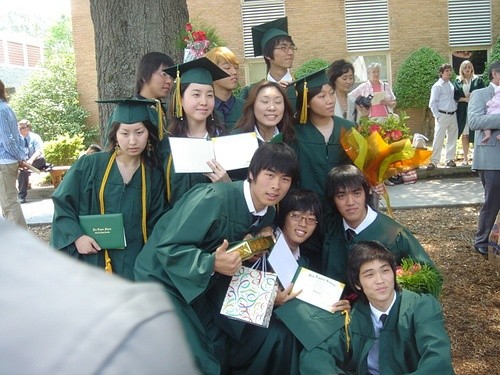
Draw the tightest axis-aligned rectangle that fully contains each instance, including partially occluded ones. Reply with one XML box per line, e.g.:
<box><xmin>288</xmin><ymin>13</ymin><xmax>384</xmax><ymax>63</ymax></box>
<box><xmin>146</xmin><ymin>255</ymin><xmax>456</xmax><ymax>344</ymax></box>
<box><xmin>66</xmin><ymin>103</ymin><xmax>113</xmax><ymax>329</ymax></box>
<box><xmin>300</xmin><ymin>164</ymin><xmax>435</xmax><ymax>296</ymax></box>
<box><xmin>466</xmin><ymin>60</ymin><xmax>500</xmax><ymax>255</ymax></box>
<box><xmin>427</xmin><ymin>63</ymin><xmax>458</xmax><ymax>170</ymax></box>
<box><xmin>300</xmin><ymin>244</ymin><xmax>456</xmax><ymax>375</ymax></box>
<box><xmin>134</xmin><ymin>141</ymin><xmax>300</xmax><ymax>375</ymax></box>
<box><xmin>227</xmin><ymin>190</ymin><xmax>351</xmax><ymax>375</ymax></box>
<box><xmin>481</xmin><ymin>85</ymin><xmax>500</xmax><ymax>142</ymax></box>
<box><xmin>453</xmin><ymin>60</ymin><xmax>486</xmax><ymax>166</ymax></box>
<box><xmin>205</xmin><ymin>46</ymin><xmax>247</xmax><ymax>135</ymax></box>
<box><xmin>347</xmin><ymin>62</ymin><xmax>397</xmax><ymax>186</ymax></box>
<box><xmin>228</xmin><ymin>79</ymin><xmax>301</xmax><ymax>157</ymax></box>
<box><xmin>240</xmin><ymin>16</ymin><xmax>301</xmax><ymax>117</ymax></box>
<box><xmin>51</xmin><ymin>98</ymin><xmax>170</xmax><ymax>282</ymax></box>
<box><xmin>285</xmin><ymin>66</ymin><xmax>417</xmax><ymax>219</ymax></box>
<box><xmin>0</xmin><ymin>218</ymin><xmax>199</xmax><ymax>375</ymax></box>
<box><xmin>104</xmin><ymin>51</ymin><xmax>175</xmax><ymax>162</ymax></box>
<box><xmin>159</xmin><ymin>56</ymin><xmax>248</xmax><ymax>208</ymax></box>
<box><xmin>18</xmin><ymin>118</ymin><xmax>46</xmax><ymax>203</ymax></box>
<box><xmin>0</xmin><ymin>80</ymin><xmax>30</xmax><ymax>228</ymax></box>
<box><xmin>328</xmin><ymin>59</ymin><xmax>360</xmax><ymax>122</ymax></box>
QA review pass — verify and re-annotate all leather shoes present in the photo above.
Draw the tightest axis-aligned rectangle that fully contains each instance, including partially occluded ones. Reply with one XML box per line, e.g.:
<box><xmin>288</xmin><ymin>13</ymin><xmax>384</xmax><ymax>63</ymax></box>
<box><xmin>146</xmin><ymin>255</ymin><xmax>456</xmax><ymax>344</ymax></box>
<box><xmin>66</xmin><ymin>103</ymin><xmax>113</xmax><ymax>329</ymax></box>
<box><xmin>474</xmin><ymin>245</ymin><xmax>488</xmax><ymax>255</ymax></box>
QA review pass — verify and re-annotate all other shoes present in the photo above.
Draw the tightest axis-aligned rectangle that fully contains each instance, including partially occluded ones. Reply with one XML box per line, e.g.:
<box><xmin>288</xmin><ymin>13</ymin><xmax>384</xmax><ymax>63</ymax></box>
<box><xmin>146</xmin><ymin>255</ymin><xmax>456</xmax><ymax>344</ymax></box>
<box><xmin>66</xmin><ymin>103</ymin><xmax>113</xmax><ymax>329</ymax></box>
<box><xmin>447</xmin><ymin>160</ymin><xmax>456</xmax><ymax>167</ymax></box>
<box><xmin>384</xmin><ymin>179</ymin><xmax>394</xmax><ymax>186</ymax></box>
<box><xmin>19</xmin><ymin>197</ymin><xmax>26</xmax><ymax>203</ymax></box>
<box><xmin>426</xmin><ymin>162</ymin><xmax>435</xmax><ymax>170</ymax></box>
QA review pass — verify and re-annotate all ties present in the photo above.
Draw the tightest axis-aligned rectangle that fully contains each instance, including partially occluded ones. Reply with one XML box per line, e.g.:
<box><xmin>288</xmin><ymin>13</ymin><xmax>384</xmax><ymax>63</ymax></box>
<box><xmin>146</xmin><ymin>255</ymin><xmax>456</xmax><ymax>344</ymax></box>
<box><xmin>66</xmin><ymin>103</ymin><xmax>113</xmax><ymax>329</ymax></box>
<box><xmin>378</xmin><ymin>313</ymin><xmax>388</xmax><ymax>325</ymax></box>
<box><xmin>250</xmin><ymin>213</ymin><xmax>259</xmax><ymax>225</ymax></box>
<box><xmin>24</xmin><ymin>137</ymin><xmax>28</xmax><ymax>148</ymax></box>
<box><xmin>345</xmin><ymin>228</ymin><xmax>356</xmax><ymax>242</ymax></box>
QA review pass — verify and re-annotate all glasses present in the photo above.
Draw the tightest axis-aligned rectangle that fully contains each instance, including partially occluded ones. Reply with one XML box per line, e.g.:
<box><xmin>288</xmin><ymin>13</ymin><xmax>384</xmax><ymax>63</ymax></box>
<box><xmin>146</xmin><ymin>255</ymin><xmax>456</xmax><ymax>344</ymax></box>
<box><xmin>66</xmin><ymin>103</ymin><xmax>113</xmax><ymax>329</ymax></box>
<box><xmin>154</xmin><ymin>71</ymin><xmax>175</xmax><ymax>81</ymax></box>
<box><xmin>273</xmin><ymin>47</ymin><xmax>297</xmax><ymax>52</ymax></box>
<box><xmin>288</xmin><ymin>212</ymin><xmax>318</xmax><ymax>226</ymax></box>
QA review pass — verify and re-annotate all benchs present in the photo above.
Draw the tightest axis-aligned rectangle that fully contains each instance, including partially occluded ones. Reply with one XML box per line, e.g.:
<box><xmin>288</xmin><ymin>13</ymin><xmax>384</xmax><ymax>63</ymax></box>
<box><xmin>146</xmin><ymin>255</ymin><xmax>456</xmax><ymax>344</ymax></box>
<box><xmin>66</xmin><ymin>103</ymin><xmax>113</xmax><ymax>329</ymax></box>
<box><xmin>29</xmin><ymin>167</ymin><xmax>70</xmax><ymax>188</ymax></box>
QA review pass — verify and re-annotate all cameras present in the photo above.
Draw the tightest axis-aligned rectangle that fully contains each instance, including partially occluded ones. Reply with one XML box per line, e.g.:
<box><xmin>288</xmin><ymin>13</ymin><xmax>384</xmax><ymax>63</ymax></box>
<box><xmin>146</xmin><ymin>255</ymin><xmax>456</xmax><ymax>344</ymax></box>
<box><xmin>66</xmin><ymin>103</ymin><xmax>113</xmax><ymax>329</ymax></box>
<box><xmin>356</xmin><ymin>93</ymin><xmax>373</xmax><ymax>108</ymax></box>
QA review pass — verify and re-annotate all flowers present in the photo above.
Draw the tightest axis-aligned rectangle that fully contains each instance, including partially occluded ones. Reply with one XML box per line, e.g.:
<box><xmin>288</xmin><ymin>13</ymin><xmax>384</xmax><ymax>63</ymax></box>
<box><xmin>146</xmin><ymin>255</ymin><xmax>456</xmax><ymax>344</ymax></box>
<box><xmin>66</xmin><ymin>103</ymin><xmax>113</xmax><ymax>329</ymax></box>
<box><xmin>181</xmin><ymin>22</ymin><xmax>224</xmax><ymax>64</ymax></box>
<box><xmin>352</xmin><ymin>112</ymin><xmax>415</xmax><ymax>144</ymax></box>
<box><xmin>394</xmin><ymin>258</ymin><xmax>442</xmax><ymax>299</ymax></box>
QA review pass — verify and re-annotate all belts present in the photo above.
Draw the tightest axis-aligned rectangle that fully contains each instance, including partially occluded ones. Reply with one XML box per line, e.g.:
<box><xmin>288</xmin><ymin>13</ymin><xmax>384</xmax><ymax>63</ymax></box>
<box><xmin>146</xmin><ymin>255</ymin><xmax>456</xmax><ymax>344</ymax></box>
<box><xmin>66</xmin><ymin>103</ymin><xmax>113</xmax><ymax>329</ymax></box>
<box><xmin>438</xmin><ymin>110</ymin><xmax>457</xmax><ymax>115</ymax></box>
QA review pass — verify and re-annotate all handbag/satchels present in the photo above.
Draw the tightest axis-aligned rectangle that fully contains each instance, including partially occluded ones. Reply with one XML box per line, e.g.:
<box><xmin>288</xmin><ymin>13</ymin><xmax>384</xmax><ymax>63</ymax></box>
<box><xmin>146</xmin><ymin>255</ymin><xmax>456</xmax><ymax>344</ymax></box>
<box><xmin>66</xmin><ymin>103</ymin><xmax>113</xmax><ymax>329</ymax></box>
<box><xmin>219</xmin><ymin>251</ymin><xmax>279</xmax><ymax>329</ymax></box>
<box><xmin>388</xmin><ymin>173</ymin><xmax>404</xmax><ymax>184</ymax></box>
<box><xmin>401</xmin><ymin>169</ymin><xmax>418</xmax><ymax>182</ymax></box>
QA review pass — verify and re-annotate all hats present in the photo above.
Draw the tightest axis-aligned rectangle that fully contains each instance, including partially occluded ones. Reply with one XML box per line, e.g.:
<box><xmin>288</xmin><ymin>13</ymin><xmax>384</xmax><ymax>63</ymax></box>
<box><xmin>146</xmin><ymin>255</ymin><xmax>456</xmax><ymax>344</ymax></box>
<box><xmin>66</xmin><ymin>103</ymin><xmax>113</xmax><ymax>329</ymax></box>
<box><xmin>95</xmin><ymin>98</ymin><xmax>164</xmax><ymax>141</ymax></box>
<box><xmin>162</xmin><ymin>57</ymin><xmax>232</xmax><ymax>118</ymax></box>
<box><xmin>251</xmin><ymin>16</ymin><xmax>290</xmax><ymax>56</ymax></box>
<box><xmin>286</xmin><ymin>66</ymin><xmax>331</xmax><ymax>124</ymax></box>
